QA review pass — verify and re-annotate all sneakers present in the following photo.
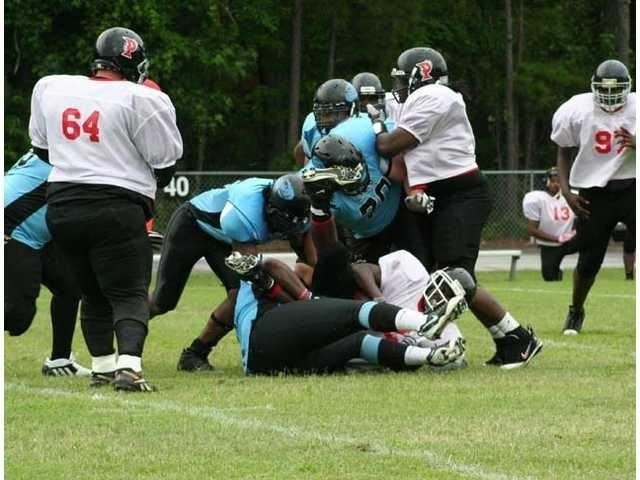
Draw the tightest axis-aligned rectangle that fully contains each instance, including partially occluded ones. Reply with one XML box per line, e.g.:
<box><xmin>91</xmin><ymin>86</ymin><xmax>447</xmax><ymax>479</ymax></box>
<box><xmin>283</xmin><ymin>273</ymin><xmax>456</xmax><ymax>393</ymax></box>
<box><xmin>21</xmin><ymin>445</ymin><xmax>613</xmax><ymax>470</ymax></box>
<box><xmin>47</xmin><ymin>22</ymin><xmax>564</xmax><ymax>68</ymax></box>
<box><xmin>498</xmin><ymin>324</ymin><xmax>544</xmax><ymax>370</ymax></box>
<box><xmin>41</xmin><ymin>351</ymin><xmax>95</xmax><ymax>378</ymax></box>
<box><xmin>300</xmin><ymin>164</ymin><xmax>362</xmax><ymax>201</ymax></box>
<box><xmin>416</xmin><ymin>295</ymin><xmax>467</xmax><ymax>341</ymax></box>
<box><xmin>562</xmin><ymin>305</ymin><xmax>586</xmax><ymax>336</ymax></box>
<box><xmin>425</xmin><ymin>336</ymin><xmax>467</xmax><ymax>367</ymax></box>
<box><xmin>111</xmin><ymin>366</ymin><xmax>160</xmax><ymax>394</ymax></box>
<box><xmin>481</xmin><ymin>336</ymin><xmax>505</xmax><ymax>367</ymax></box>
<box><xmin>224</xmin><ymin>250</ymin><xmax>261</xmax><ymax>280</ymax></box>
<box><xmin>173</xmin><ymin>347</ymin><xmax>215</xmax><ymax>372</ymax></box>
<box><xmin>89</xmin><ymin>370</ymin><xmax>116</xmax><ymax>388</ymax></box>
<box><xmin>260</xmin><ymin>272</ymin><xmax>281</xmax><ymax>298</ymax></box>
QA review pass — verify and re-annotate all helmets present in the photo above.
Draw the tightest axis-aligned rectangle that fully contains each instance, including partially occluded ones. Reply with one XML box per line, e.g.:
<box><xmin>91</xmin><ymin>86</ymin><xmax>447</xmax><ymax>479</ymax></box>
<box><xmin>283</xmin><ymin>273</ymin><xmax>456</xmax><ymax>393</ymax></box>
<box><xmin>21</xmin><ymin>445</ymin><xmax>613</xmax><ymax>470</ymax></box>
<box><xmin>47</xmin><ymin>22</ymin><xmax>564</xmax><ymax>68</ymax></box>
<box><xmin>542</xmin><ymin>165</ymin><xmax>560</xmax><ymax>182</ymax></box>
<box><xmin>444</xmin><ymin>266</ymin><xmax>477</xmax><ymax>307</ymax></box>
<box><xmin>91</xmin><ymin>25</ymin><xmax>149</xmax><ymax>84</ymax></box>
<box><xmin>391</xmin><ymin>46</ymin><xmax>449</xmax><ymax>91</ymax></box>
<box><xmin>312</xmin><ymin>78</ymin><xmax>359</xmax><ymax>119</ymax></box>
<box><xmin>351</xmin><ymin>72</ymin><xmax>386</xmax><ymax>98</ymax></box>
<box><xmin>591</xmin><ymin>58</ymin><xmax>631</xmax><ymax>92</ymax></box>
<box><xmin>311</xmin><ymin>134</ymin><xmax>371</xmax><ymax>196</ymax></box>
<box><xmin>265</xmin><ymin>172</ymin><xmax>313</xmax><ymax>224</ymax></box>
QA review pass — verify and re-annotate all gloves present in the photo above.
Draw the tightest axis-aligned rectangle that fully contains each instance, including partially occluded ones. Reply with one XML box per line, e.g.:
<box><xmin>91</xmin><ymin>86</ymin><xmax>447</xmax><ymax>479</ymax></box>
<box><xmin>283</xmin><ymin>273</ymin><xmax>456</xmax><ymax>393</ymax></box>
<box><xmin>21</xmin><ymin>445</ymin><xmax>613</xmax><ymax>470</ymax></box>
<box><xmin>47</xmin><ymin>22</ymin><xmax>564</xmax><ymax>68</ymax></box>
<box><xmin>365</xmin><ymin>103</ymin><xmax>388</xmax><ymax>135</ymax></box>
<box><xmin>404</xmin><ymin>192</ymin><xmax>437</xmax><ymax>216</ymax></box>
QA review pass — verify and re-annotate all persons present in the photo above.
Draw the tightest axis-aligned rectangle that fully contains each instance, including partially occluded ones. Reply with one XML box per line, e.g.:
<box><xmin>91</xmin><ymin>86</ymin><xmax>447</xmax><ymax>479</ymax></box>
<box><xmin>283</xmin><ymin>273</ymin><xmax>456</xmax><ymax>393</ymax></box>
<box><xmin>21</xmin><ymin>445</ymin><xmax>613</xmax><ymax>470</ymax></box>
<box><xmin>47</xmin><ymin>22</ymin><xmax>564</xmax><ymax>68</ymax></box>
<box><xmin>146</xmin><ymin>46</ymin><xmax>543</xmax><ymax>377</ymax></box>
<box><xmin>522</xmin><ymin>167</ymin><xmax>580</xmax><ymax>282</ymax></box>
<box><xmin>4</xmin><ymin>78</ymin><xmax>161</xmax><ymax>377</ymax></box>
<box><xmin>550</xmin><ymin>57</ymin><xmax>635</xmax><ymax>336</ymax></box>
<box><xmin>25</xmin><ymin>26</ymin><xmax>184</xmax><ymax>393</ymax></box>
